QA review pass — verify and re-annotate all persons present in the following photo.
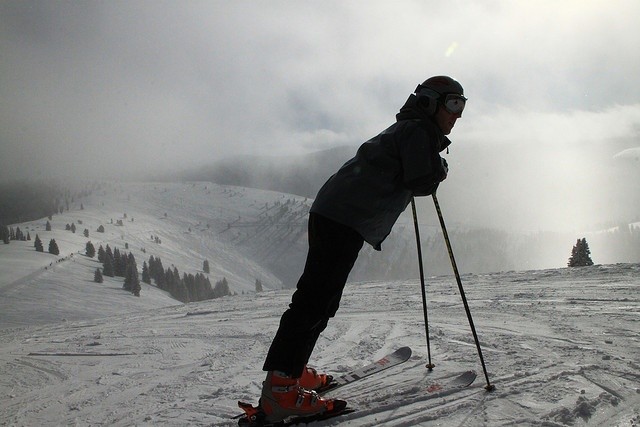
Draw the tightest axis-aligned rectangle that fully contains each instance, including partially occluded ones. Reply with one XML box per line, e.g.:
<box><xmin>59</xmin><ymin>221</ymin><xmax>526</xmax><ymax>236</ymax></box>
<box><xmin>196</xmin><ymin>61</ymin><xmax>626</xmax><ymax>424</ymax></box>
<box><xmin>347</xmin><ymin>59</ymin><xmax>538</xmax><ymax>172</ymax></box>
<box><xmin>254</xmin><ymin>74</ymin><xmax>468</xmax><ymax>422</ymax></box>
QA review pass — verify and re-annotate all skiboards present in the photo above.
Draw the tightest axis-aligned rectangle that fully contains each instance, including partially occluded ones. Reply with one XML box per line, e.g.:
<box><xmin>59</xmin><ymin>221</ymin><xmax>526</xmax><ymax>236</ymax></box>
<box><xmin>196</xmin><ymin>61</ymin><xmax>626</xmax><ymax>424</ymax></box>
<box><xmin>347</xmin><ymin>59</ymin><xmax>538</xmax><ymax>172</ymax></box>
<box><xmin>230</xmin><ymin>346</ymin><xmax>478</xmax><ymax>427</ymax></box>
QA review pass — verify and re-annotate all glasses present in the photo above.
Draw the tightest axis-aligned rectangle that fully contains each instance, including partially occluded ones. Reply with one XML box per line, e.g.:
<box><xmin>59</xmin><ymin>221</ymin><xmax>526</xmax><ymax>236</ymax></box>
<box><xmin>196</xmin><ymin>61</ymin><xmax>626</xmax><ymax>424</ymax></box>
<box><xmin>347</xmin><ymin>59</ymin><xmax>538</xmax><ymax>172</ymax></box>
<box><xmin>439</xmin><ymin>92</ymin><xmax>467</xmax><ymax>119</ymax></box>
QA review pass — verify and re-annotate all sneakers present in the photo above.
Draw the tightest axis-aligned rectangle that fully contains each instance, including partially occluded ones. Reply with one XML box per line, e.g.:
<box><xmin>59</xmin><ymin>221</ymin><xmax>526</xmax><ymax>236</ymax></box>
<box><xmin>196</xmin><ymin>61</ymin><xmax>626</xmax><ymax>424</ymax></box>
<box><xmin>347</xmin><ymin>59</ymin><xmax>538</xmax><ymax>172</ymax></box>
<box><xmin>300</xmin><ymin>366</ymin><xmax>332</xmax><ymax>391</ymax></box>
<box><xmin>260</xmin><ymin>370</ymin><xmax>347</xmax><ymax>420</ymax></box>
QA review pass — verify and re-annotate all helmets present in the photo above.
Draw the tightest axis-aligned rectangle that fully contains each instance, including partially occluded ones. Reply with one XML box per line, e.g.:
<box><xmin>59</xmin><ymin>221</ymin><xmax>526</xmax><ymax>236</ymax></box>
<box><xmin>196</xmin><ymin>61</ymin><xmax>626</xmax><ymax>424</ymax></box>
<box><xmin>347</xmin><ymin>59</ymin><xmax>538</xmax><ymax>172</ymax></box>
<box><xmin>416</xmin><ymin>75</ymin><xmax>464</xmax><ymax>113</ymax></box>
